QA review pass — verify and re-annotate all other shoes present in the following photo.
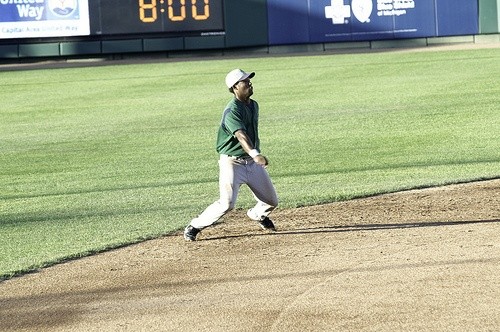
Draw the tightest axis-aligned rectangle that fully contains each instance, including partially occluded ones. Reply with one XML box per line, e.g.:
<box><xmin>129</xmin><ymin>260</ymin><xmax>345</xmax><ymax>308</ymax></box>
<box><xmin>184</xmin><ymin>224</ymin><xmax>199</xmax><ymax>242</ymax></box>
<box><xmin>259</xmin><ymin>217</ymin><xmax>275</xmax><ymax>230</ymax></box>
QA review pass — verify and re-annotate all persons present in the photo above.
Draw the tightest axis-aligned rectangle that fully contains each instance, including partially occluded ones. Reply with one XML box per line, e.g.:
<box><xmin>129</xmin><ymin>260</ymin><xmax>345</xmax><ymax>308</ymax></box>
<box><xmin>183</xmin><ymin>69</ymin><xmax>278</xmax><ymax>241</ymax></box>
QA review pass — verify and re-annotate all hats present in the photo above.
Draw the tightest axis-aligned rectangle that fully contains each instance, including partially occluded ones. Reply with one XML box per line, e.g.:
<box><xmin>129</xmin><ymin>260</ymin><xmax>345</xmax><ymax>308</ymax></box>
<box><xmin>225</xmin><ymin>68</ymin><xmax>256</xmax><ymax>88</ymax></box>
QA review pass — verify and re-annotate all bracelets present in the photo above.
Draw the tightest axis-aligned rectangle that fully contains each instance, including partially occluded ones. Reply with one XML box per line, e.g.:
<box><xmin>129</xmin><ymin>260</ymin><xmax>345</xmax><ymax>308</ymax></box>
<box><xmin>248</xmin><ymin>149</ymin><xmax>260</xmax><ymax>159</ymax></box>
